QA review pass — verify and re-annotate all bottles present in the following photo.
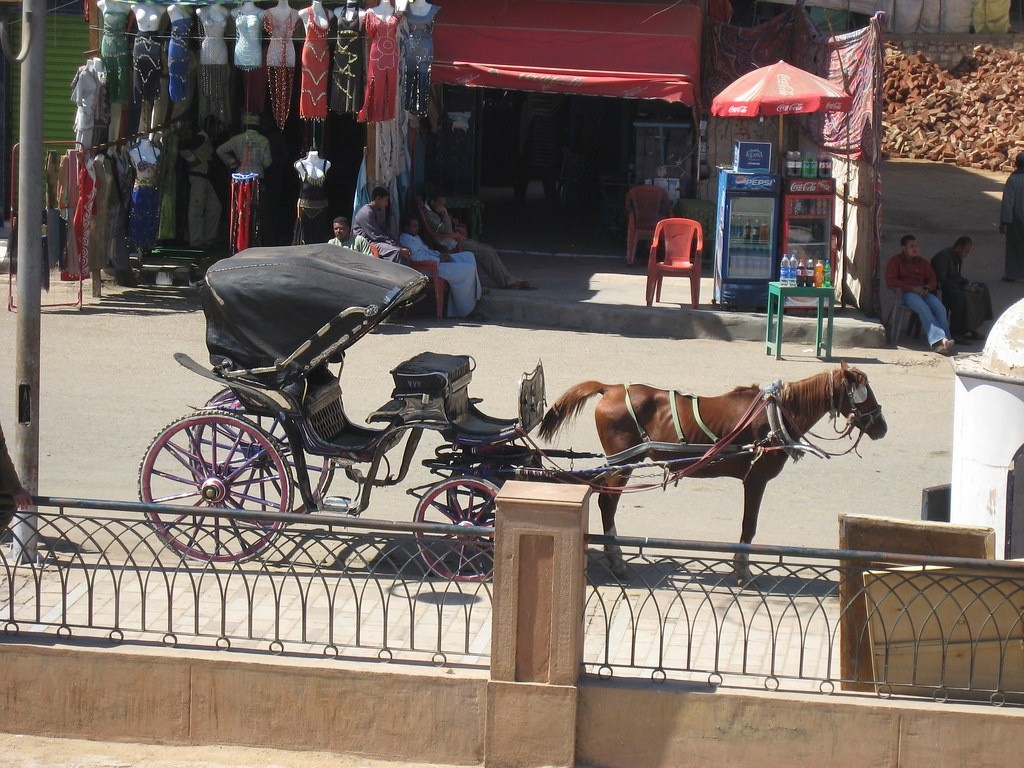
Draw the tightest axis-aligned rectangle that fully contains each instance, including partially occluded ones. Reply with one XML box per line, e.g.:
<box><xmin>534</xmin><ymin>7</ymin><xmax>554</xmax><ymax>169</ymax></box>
<box><xmin>791</xmin><ymin>199</ymin><xmax>828</xmax><ymax>215</ymax></box>
<box><xmin>780</xmin><ymin>254</ymin><xmax>831</xmax><ymax>288</ymax></box>
<box><xmin>731</xmin><ymin>215</ymin><xmax>769</xmax><ymax>242</ymax></box>
<box><xmin>785</xmin><ymin>151</ymin><xmax>833</xmax><ymax>178</ymax></box>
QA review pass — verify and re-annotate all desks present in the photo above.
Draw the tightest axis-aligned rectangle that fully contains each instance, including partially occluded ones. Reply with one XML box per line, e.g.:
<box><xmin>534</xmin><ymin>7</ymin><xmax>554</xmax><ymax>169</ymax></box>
<box><xmin>766</xmin><ymin>282</ymin><xmax>836</xmax><ymax>360</ymax></box>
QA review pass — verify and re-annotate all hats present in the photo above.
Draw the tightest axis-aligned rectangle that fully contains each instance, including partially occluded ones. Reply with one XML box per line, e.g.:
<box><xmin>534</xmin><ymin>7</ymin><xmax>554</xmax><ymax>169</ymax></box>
<box><xmin>241</xmin><ymin>111</ymin><xmax>260</xmax><ymax>125</ymax></box>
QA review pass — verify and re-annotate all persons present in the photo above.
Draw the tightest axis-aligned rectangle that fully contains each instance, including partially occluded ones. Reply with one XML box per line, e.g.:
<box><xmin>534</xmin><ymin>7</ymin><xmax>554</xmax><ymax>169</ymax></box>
<box><xmin>71</xmin><ymin>1</ymin><xmax>441</xmax><ymax>252</ymax></box>
<box><xmin>216</xmin><ymin>112</ymin><xmax>272</xmax><ymax>199</ymax></box>
<box><xmin>328</xmin><ymin>216</ymin><xmax>375</xmax><ymax>257</ymax></box>
<box><xmin>420</xmin><ymin>190</ymin><xmax>537</xmax><ymax>289</ymax></box>
<box><xmin>355</xmin><ymin>187</ymin><xmax>408</xmax><ymax>264</ymax></box>
<box><xmin>177</xmin><ymin>115</ymin><xmax>223</xmax><ymax>248</ymax></box>
<box><xmin>886</xmin><ymin>235</ymin><xmax>954</xmax><ymax>354</ymax></box>
<box><xmin>400</xmin><ymin>215</ymin><xmax>482</xmax><ymax>319</ymax></box>
<box><xmin>932</xmin><ymin>236</ymin><xmax>993</xmax><ymax>346</ymax></box>
<box><xmin>0</xmin><ymin>423</ymin><xmax>33</xmax><ymax>531</ymax></box>
<box><xmin>999</xmin><ymin>154</ymin><xmax>1024</xmax><ymax>282</ymax></box>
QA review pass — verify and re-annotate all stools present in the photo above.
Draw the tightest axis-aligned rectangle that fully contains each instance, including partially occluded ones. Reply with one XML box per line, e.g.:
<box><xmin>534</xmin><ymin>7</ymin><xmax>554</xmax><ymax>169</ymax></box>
<box><xmin>387</xmin><ymin>350</ymin><xmax>477</xmax><ymax>397</ymax></box>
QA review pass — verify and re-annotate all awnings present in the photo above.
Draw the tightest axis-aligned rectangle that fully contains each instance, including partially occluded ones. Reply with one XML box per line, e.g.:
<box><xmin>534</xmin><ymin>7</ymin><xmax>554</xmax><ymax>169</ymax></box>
<box><xmin>431</xmin><ymin>0</ymin><xmax>702</xmax><ymax>108</ymax></box>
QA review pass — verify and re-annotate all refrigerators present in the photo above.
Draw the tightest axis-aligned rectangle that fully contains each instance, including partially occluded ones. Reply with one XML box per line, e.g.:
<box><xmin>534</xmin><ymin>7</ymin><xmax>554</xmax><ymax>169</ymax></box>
<box><xmin>712</xmin><ymin>168</ymin><xmax>780</xmax><ymax>311</ymax></box>
<box><xmin>781</xmin><ymin>177</ymin><xmax>836</xmax><ymax>313</ymax></box>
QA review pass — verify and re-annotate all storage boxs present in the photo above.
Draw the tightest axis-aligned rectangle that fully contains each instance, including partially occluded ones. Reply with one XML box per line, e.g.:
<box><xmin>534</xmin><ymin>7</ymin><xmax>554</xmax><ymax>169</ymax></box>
<box><xmin>734</xmin><ymin>139</ymin><xmax>771</xmax><ymax>175</ymax></box>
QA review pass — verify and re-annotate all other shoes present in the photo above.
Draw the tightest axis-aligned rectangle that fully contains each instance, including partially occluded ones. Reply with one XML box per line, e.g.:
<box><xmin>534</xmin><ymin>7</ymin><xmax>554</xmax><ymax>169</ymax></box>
<box><xmin>1002</xmin><ymin>277</ymin><xmax>1015</xmax><ymax>282</ymax></box>
<box><xmin>952</xmin><ymin>336</ymin><xmax>971</xmax><ymax>346</ymax></box>
<box><xmin>934</xmin><ymin>339</ymin><xmax>954</xmax><ymax>356</ymax></box>
<box><xmin>968</xmin><ymin>331</ymin><xmax>984</xmax><ymax>340</ymax></box>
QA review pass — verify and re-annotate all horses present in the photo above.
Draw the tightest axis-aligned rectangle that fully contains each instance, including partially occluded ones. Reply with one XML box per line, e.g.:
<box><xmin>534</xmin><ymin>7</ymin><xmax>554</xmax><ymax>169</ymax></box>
<box><xmin>537</xmin><ymin>356</ymin><xmax>888</xmax><ymax>587</ymax></box>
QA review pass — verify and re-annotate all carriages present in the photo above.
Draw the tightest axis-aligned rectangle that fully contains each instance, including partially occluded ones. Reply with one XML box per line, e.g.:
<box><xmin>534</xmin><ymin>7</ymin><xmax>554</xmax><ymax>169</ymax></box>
<box><xmin>137</xmin><ymin>242</ymin><xmax>891</xmax><ymax>583</ymax></box>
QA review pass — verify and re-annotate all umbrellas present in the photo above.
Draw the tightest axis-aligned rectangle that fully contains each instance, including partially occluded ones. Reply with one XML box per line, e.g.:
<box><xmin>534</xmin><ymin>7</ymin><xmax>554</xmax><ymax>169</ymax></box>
<box><xmin>711</xmin><ymin>60</ymin><xmax>853</xmax><ymax>155</ymax></box>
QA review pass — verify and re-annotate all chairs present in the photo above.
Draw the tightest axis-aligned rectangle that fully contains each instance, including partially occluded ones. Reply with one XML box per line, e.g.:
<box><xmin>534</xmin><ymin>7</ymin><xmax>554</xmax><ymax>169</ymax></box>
<box><xmin>291</xmin><ymin>350</ymin><xmax>344</xmax><ymax>418</ymax></box>
<box><xmin>414</xmin><ymin>196</ymin><xmax>467</xmax><ymax>251</ymax></box>
<box><xmin>400</xmin><ymin>248</ymin><xmax>447</xmax><ymax>321</ymax></box>
<box><xmin>646</xmin><ymin>217</ymin><xmax>703</xmax><ymax>308</ymax></box>
<box><xmin>369</xmin><ymin>243</ymin><xmax>382</xmax><ymax>260</ymax></box>
<box><xmin>881</xmin><ymin>284</ymin><xmax>935</xmax><ymax>345</ymax></box>
<box><xmin>624</xmin><ymin>185</ymin><xmax>675</xmax><ymax>264</ymax></box>
